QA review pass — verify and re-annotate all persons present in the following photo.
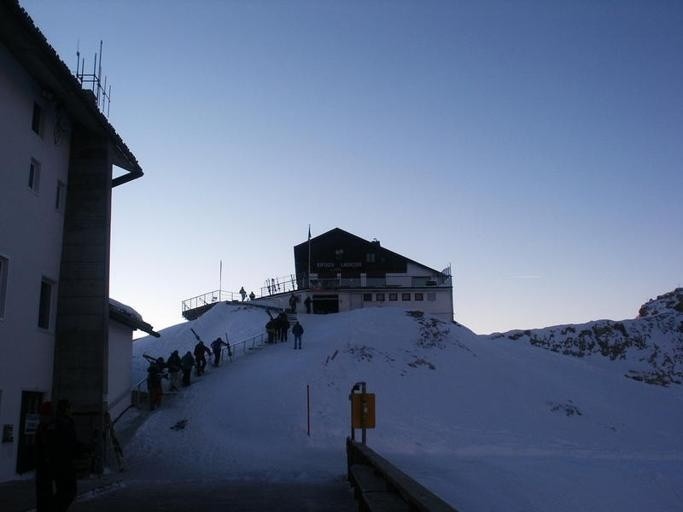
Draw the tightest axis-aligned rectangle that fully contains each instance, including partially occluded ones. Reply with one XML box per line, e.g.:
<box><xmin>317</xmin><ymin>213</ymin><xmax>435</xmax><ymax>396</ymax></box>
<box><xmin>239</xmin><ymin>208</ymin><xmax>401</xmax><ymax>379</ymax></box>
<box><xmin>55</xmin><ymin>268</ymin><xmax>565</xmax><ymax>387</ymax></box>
<box><xmin>193</xmin><ymin>341</ymin><xmax>211</xmax><ymax>376</ymax></box>
<box><xmin>303</xmin><ymin>296</ymin><xmax>312</xmax><ymax>314</ymax></box>
<box><xmin>179</xmin><ymin>350</ymin><xmax>194</xmax><ymax>388</ymax></box>
<box><xmin>288</xmin><ymin>292</ymin><xmax>297</xmax><ymax>313</ymax></box>
<box><xmin>248</xmin><ymin>291</ymin><xmax>255</xmax><ymax>301</ymax></box>
<box><xmin>239</xmin><ymin>285</ymin><xmax>246</xmax><ymax>301</ymax></box>
<box><xmin>167</xmin><ymin>349</ymin><xmax>181</xmax><ymax>392</ymax></box>
<box><xmin>210</xmin><ymin>336</ymin><xmax>230</xmax><ymax>366</ymax></box>
<box><xmin>52</xmin><ymin>397</ymin><xmax>78</xmax><ymax>511</ymax></box>
<box><xmin>33</xmin><ymin>399</ymin><xmax>56</xmax><ymax>511</ymax></box>
<box><xmin>146</xmin><ymin>356</ymin><xmax>169</xmax><ymax>411</ymax></box>
<box><xmin>264</xmin><ymin>311</ymin><xmax>290</xmax><ymax>344</ymax></box>
<box><xmin>290</xmin><ymin>320</ymin><xmax>304</xmax><ymax>350</ymax></box>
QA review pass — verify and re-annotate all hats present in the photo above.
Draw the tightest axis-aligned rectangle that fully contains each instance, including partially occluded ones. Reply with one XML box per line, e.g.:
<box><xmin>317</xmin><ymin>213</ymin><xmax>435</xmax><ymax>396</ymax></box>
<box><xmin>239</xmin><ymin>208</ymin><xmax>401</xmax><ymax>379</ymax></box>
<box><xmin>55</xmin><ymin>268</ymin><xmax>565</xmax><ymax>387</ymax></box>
<box><xmin>40</xmin><ymin>399</ymin><xmax>54</xmax><ymax>416</ymax></box>
